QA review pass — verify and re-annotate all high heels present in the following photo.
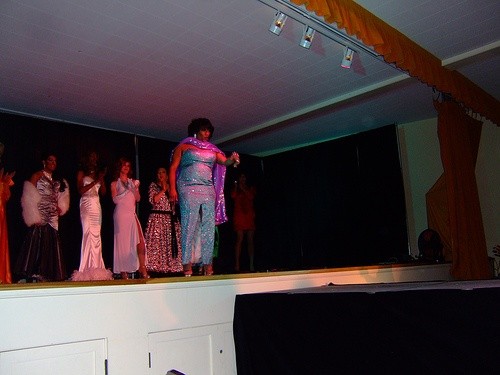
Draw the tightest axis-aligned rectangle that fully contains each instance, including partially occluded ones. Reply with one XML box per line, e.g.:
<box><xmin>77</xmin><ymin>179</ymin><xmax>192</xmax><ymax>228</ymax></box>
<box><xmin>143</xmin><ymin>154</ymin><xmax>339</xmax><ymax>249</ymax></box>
<box><xmin>138</xmin><ymin>265</ymin><xmax>150</xmax><ymax>279</ymax></box>
<box><xmin>183</xmin><ymin>265</ymin><xmax>192</xmax><ymax>277</ymax></box>
<box><xmin>199</xmin><ymin>265</ymin><xmax>213</xmax><ymax>276</ymax></box>
<box><xmin>120</xmin><ymin>272</ymin><xmax>129</xmax><ymax>281</ymax></box>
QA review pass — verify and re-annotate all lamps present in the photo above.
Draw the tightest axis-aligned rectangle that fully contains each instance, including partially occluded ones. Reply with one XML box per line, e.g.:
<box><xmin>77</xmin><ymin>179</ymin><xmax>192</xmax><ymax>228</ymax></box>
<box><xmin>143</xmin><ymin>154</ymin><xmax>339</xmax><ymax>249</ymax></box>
<box><xmin>340</xmin><ymin>46</ymin><xmax>354</xmax><ymax>69</ymax></box>
<box><xmin>269</xmin><ymin>11</ymin><xmax>288</xmax><ymax>37</ymax></box>
<box><xmin>299</xmin><ymin>26</ymin><xmax>317</xmax><ymax>49</ymax></box>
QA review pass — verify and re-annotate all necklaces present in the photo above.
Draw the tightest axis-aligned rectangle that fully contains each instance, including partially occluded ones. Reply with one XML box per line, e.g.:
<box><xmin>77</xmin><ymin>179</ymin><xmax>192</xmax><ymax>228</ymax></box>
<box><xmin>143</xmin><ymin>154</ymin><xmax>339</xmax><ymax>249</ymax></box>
<box><xmin>41</xmin><ymin>169</ymin><xmax>52</xmax><ymax>181</ymax></box>
<box><xmin>88</xmin><ymin>168</ymin><xmax>96</xmax><ymax>173</ymax></box>
<box><xmin>120</xmin><ymin>179</ymin><xmax>129</xmax><ymax>191</ymax></box>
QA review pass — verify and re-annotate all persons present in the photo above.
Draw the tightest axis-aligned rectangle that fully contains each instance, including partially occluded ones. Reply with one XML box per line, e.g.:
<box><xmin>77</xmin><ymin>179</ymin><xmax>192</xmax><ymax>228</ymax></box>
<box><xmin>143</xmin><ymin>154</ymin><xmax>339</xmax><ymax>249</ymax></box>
<box><xmin>169</xmin><ymin>117</ymin><xmax>240</xmax><ymax>276</ymax></box>
<box><xmin>69</xmin><ymin>150</ymin><xmax>114</xmax><ymax>280</ymax></box>
<box><xmin>231</xmin><ymin>174</ymin><xmax>256</xmax><ymax>273</ymax></box>
<box><xmin>16</xmin><ymin>154</ymin><xmax>70</xmax><ymax>281</ymax></box>
<box><xmin>0</xmin><ymin>166</ymin><xmax>16</xmax><ymax>284</ymax></box>
<box><xmin>137</xmin><ymin>167</ymin><xmax>183</xmax><ymax>279</ymax></box>
<box><xmin>197</xmin><ymin>226</ymin><xmax>219</xmax><ymax>276</ymax></box>
<box><xmin>111</xmin><ymin>159</ymin><xmax>150</xmax><ymax>280</ymax></box>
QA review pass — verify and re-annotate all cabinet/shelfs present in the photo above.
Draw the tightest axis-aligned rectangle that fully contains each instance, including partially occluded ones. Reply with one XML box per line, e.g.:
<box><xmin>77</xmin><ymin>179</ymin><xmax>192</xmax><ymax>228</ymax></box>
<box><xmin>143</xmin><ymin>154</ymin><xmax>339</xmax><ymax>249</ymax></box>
<box><xmin>0</xmin><ymin>321</ymin><xmax>237</xmax><ymax>375</ymax></box>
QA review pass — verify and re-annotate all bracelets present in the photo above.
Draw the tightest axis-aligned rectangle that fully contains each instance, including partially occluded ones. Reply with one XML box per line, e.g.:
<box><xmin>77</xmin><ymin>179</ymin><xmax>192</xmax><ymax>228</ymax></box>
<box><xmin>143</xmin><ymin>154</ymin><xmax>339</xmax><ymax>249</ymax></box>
<box><xmin>94</xmin><ymin>180</ymin><xmax>96</xmax><ymax>185</ymax></box>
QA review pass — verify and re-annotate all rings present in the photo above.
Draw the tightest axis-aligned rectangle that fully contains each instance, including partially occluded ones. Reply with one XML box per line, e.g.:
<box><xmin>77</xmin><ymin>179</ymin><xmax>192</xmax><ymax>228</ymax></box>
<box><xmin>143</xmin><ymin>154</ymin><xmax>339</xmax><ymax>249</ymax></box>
<box><xmin>171</xmin><ymin>199</ymin><xmax>173</xmax><ymax>202</ymax></box>
